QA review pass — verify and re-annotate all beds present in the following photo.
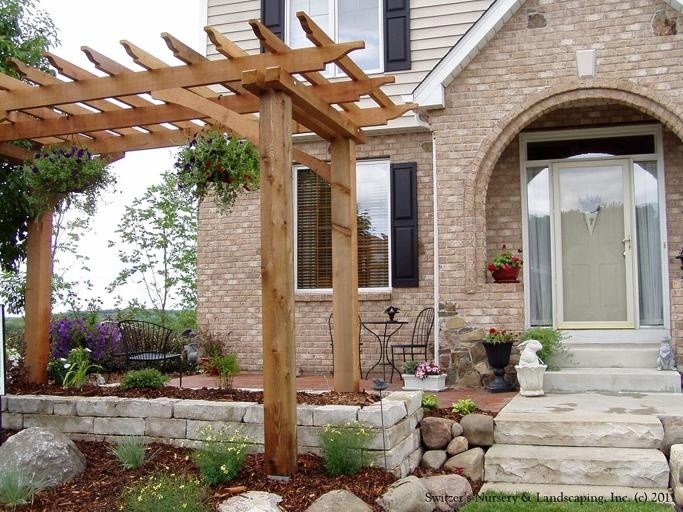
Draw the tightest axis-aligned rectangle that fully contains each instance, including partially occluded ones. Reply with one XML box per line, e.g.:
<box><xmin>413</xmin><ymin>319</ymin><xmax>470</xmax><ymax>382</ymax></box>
<box><xmin>361</xmin><ymin>321</ymin><xmax>409</xmax><ymax>383</ymax></box>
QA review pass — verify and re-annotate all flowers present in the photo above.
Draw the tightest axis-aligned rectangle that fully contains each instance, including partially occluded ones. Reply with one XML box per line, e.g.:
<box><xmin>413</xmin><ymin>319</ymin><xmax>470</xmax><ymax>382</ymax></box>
<box><xmin>493</xmin><ymin>251</ymin><xmax>522</xmax><ymax>270</ymax></box>
<box><xmin>402</xmin><ymin>360</ymin><xmax>440</xmax><ymax>380</ymax></box>
<box><xmin>484</xmin><ymin>328</ymin><xmax>515</xmax><ymax>344</ymax></box>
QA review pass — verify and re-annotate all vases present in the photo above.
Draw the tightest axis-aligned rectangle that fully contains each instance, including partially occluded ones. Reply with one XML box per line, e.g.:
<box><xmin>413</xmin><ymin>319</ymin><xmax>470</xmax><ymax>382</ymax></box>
<box><xmin>487</xmin><ymin>264</ymin><xmax>521</xmax><ymax>283</ymax></box>
<box><xmin>402</xmin><ymin>373</ymin><xmax>448</xmax><ymax>392</ymax></box>
<box><xmin>483</xmin><ymin>341</ymin><xmax>517</xmax><ymax>393</ymax></box>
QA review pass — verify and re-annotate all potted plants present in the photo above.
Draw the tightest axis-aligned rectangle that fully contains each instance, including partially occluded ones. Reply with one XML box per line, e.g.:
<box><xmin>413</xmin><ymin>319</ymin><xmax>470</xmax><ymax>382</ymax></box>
<box><xmin>201</xmin><ymin>343</ymin><xmax>222</xmax><ymax>376</ymax></box>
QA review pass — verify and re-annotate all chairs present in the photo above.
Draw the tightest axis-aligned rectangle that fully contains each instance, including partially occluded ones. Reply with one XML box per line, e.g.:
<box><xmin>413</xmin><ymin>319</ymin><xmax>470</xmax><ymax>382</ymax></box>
<box><xmin>390</xmin><ymin>307</ymin><xmax>434</xmax><ymax>383</ymax></box>
<box><xmin>329</xmin><ymin>312</ymin><xmax>362</xmax><ymax>379</ymax></box>
<box><xmin>118</xmin><ymin>320</ymin><xmax>182</xmax><ymax>386</ymax></box>
